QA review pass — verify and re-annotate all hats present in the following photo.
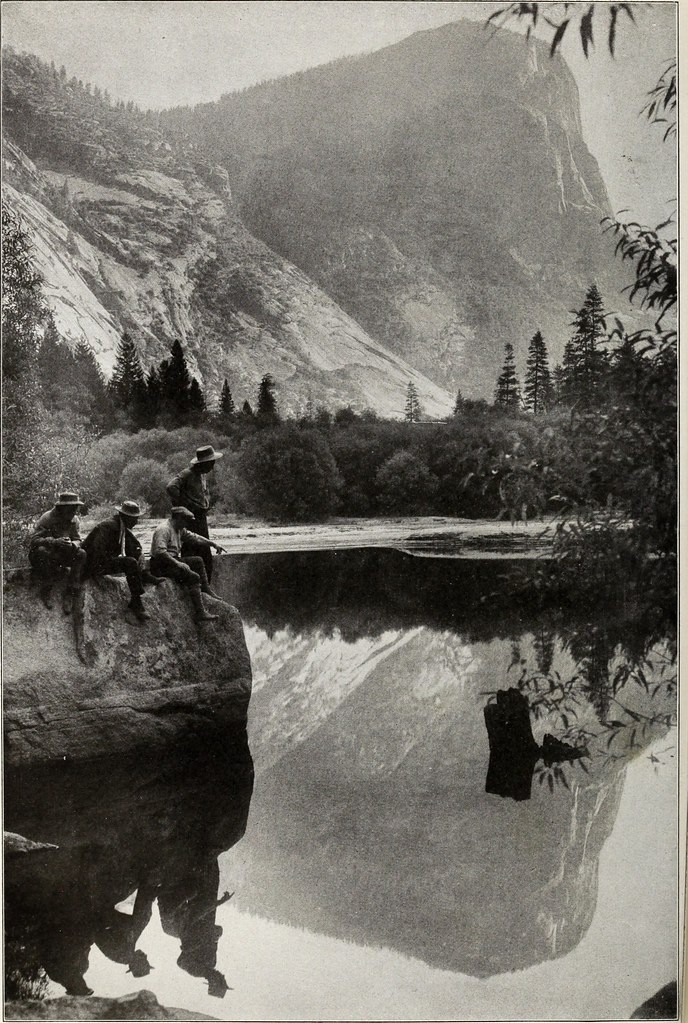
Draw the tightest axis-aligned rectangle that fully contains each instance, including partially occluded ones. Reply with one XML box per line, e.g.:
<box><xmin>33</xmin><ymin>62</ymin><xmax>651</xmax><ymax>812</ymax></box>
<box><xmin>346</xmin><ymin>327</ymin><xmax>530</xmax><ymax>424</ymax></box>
<box><xmin>114</xmin><ymin>500</ymin><xmax>144</xmax><ymax>516</ymax></box>
<box><xmin>171</xmin><ymin>506</ymin><xmax>196</xmax><ymax>520</ymax></box>
<box><xmin>53</xmin><ymin>492</ymin><xmax>84</xmax><ymax>506</ymax></box>
<box><xmin>190</xmin><ymin>445</ymin><xmax>223</xmax><ymax>464</ymax></box>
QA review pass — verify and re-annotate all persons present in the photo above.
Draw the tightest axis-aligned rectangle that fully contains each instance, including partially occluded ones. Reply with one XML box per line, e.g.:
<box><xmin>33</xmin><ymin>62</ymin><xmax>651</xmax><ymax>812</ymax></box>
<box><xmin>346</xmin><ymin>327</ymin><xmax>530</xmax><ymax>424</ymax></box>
<box><xmin>30</xmin><ymin>492</ymin><xmax>87</xmax><ymax>614</ymax></box>
<box><xmin>34</xmin><ymin>850</ymin><xmax>237</xmax><ymax>1002</ymax></box>
<box><xmin>151</xmin><ymin>506</ymin><xmax>227</xmax><ymax>620</ymax></box>
<box><xmin>81</xmin><ymin>501</ymin><xmax>166</xmax><ymax>620</ymax></box>
<box><xmin>167</xmin><ymin>446</ymin><xmax>223</xmax><ymax>591</ymax></box>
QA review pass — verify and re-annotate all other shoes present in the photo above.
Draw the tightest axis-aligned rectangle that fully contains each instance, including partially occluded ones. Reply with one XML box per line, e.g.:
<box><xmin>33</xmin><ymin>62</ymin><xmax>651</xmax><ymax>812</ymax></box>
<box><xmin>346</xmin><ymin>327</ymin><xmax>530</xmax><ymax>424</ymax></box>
<box><xmin>39</xmin><ymin>590</ymin><xmax>53</xmax><ymax>608</ymax></box>
<box><xmin>142</xmin><ymin>571</ymin><xmax>166</xmax><ymax>583</ymax></box>
<box><xmin>196</xmin><ymin>611</ymin><xmax>219</xmax><ymax>620</ymax></box>
<box><xmin>128</xmin><ymin>595</ymin><xmax>151</xmax><ymax>619</ymax></box>
<box><xmin>63</xmin><ymin>594</ymin><xmax>75</xmax><ymax>614</ymax></box>
<box><xmin>207</xmin><ymin>590</ymin><xmax>221</xmax><ymax>599</ymax></box>
